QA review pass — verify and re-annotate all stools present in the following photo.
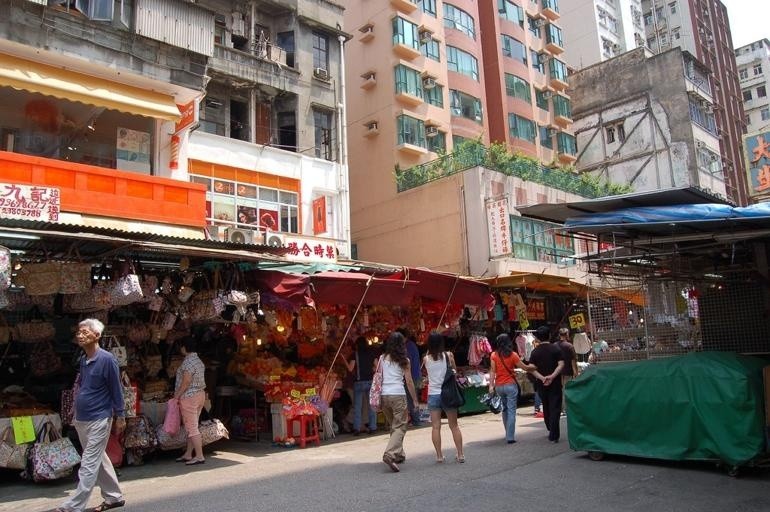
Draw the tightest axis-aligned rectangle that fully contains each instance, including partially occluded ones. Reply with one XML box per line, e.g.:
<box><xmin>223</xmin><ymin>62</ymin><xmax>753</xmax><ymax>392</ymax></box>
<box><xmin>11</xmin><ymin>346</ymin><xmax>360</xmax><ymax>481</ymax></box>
<box><xmin>288</xmin><ymin>413</ymin><xmax>321</xmax><ymax>450</ymax></box>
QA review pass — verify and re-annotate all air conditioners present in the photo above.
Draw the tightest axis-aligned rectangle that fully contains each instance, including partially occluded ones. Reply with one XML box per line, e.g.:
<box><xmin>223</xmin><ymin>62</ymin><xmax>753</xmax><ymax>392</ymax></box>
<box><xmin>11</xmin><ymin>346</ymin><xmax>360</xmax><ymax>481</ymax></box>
<box><xmin>362</xmin><ymin>27</ymin><xmax>378</xmax><ymax>132</ymax></box>
<box><xmin>535</xmin><ymin>18</ymin><xmax>558</xmax><ymax>138</ymax></box>
<box><xmin>420</xmin><ymin>32</ymin><xmax>438</xmax><ymax>136</ymax></box>
<box><xmin>315</xmin><ymin>67</ymin><xmax>330</xmax><ymax>81</ymax></box>
<box><xmin>223</xmin><ymin>227</ymin><xmax>254</xmax><ymax>245</ymax></box>
<box><xmin>265</xmin><ymin>231</ymin><xmax>284</xmax><ymax>247</ymax></box>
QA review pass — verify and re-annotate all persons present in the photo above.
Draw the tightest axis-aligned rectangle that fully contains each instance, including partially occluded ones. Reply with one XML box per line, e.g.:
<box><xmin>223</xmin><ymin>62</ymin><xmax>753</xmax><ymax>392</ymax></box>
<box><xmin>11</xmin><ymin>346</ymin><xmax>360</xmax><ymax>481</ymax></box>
<box><xmin>45</xmin><ymin>318</ymin><xmax>129</xmax><ymax>512</ymax></box>
<box><xmin>237</xmin><ymin>210</ymin><xmax>257</xmax><ymax>229</ymax></box>
<box><xmin>340</xmin><ymin>326</ymin><xmax>593</xmax><ymax>472</ymax></box>
<box><xmin>173</xmin><ymin>336</ymin><xmax>210</xmax><ymax>465</ymax></box>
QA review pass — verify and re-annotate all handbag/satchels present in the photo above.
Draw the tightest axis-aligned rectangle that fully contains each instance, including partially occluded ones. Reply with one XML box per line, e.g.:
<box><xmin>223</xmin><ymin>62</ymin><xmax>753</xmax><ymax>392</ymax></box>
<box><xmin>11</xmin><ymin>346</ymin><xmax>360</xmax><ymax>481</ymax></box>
<box><xmin>0</xmin><ymin>246</ymin><xmax>260</xmax><ymax>344</ymax></box>
<box><xmin>369</xmin><ymin>372</ymin><xmax>383</xmax><ymax>411</ymax></box>
<box><xmin>0</xmin><ymin>421</ymin><xmax>82</xmax><ymax>483</ymax></box>
<box><xmin>441</xmin><ymin>369</ymin><xmax>466</xmax><ymax>408</ymax></box>
<box><xmin>0</xmin><ymin>351</ymin><xmax>82</xmax><ymax>422</ymax></box>
<box><xmin>495</xmin><ymin>294</ymin><xmax>529</xmax><ymax>330</ymax></box>
<box><xmin>106</xmin><ymin>346</ymin><xmax>229</xmax><ymax>465</ymax></box>
<box><xmin>515</xmin><ymin>382</ymin><xmax>522</xmax><ymax>397</ymax></box>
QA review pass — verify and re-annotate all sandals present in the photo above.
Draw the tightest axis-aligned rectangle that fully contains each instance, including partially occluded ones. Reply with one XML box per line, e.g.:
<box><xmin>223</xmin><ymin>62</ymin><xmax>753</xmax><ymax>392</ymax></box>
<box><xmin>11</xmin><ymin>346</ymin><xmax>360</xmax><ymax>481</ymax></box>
<box><xmin>436</xmin><ymin>456</ymin><xmax>446</xmax><ymax>464</ymax></box>
<box><xmin>455</xmin><ymin>454</ymin><xmax>465</xmax><ymax>463</ymax></box>
<box><xmin>93</xmin><ymin>500</ymin><xmax>125</xmax><ymax>512</ymax></box>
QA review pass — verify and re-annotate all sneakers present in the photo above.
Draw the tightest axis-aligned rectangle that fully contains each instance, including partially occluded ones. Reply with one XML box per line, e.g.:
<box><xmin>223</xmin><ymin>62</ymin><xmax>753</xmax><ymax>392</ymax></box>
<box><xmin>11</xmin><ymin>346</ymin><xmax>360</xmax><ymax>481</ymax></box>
<box><xmin>560</xmin><ymin>412</ymin><xmax>566</xmax><ymax>415</ymax></box>
<box><xmin>383</xmin><ymin>455</ymin><xmax>403</xmax><ymax>472</ymax></box>
<box><xmin>535</xmin><ymin>408</ymin><xmax>540</xmax><ymax>414</ymax></box>
<box><xmin>352</xmin><ymin>430</ymin><xmax>378</xmax><ymax>436</ymax></box>
